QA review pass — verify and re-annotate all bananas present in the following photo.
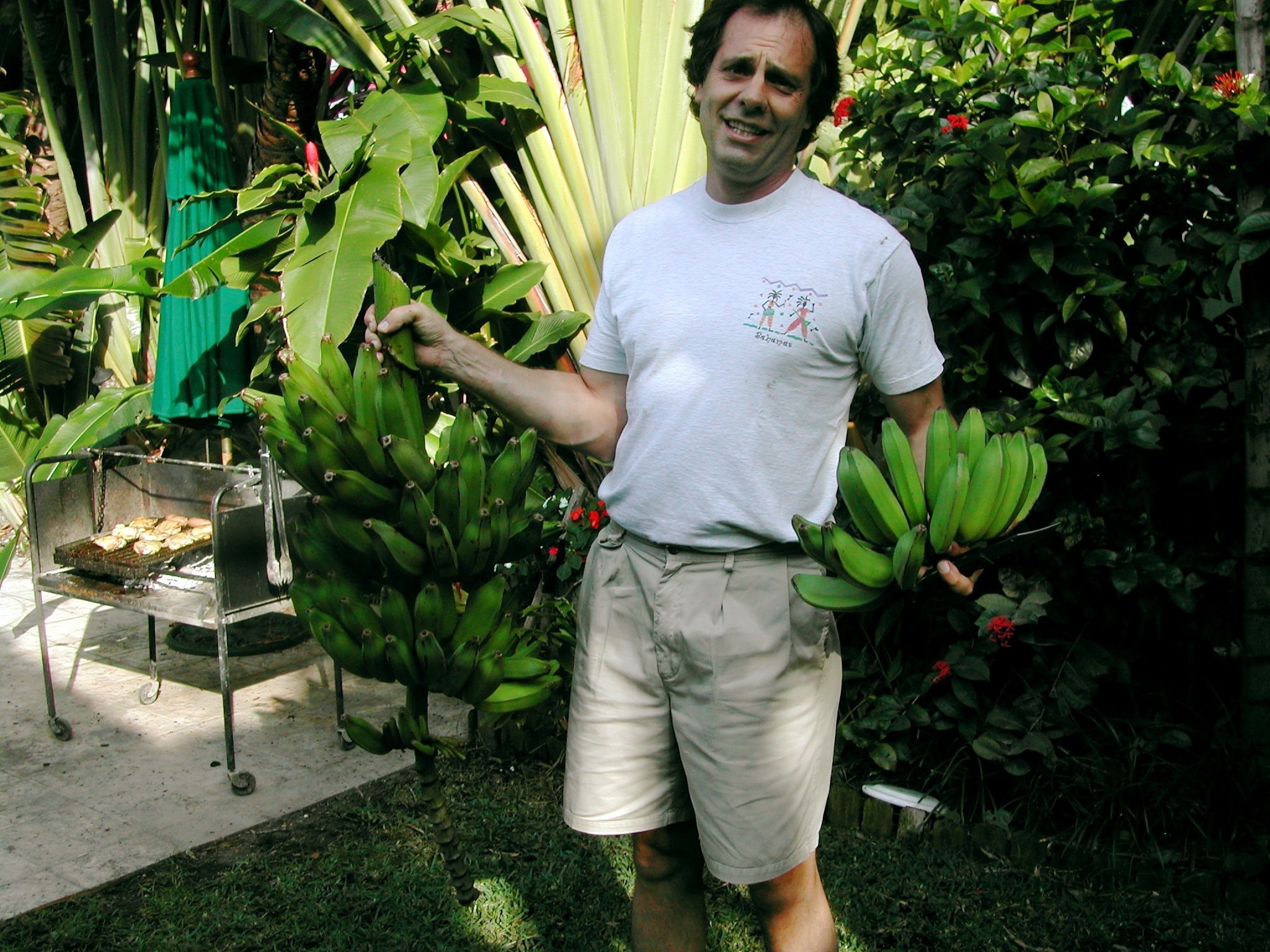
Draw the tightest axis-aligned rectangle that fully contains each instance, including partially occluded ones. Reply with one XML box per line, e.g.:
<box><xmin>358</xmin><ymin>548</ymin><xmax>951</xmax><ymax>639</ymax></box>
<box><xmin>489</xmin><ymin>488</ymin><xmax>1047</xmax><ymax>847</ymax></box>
<box><xmin>791</xmin><ymin>406</ymin><xmax>1049</xmax><ymax>610</ymax></box>
<box><xmin>241</xmin><ymin>333</ymin><xmax>564</xmax><ymax>761</ymax></box>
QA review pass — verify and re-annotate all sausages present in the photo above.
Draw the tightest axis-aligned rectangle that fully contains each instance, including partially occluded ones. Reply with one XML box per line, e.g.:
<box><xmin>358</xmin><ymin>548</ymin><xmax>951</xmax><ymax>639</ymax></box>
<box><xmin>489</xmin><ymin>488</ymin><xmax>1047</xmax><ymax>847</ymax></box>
<box><xmin>165</xmin><ymin>514</ymin><xmax>211</xmax><ymax>528</ymax></box>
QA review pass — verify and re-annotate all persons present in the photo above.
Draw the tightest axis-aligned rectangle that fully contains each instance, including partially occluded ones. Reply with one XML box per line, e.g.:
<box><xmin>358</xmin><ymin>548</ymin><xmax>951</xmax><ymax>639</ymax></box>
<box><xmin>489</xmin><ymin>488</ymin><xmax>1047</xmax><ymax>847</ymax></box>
<box><xmin>361</xmin><ymin>1</ymin><xmax>986</xmax><ymax>951</ymax></box>
